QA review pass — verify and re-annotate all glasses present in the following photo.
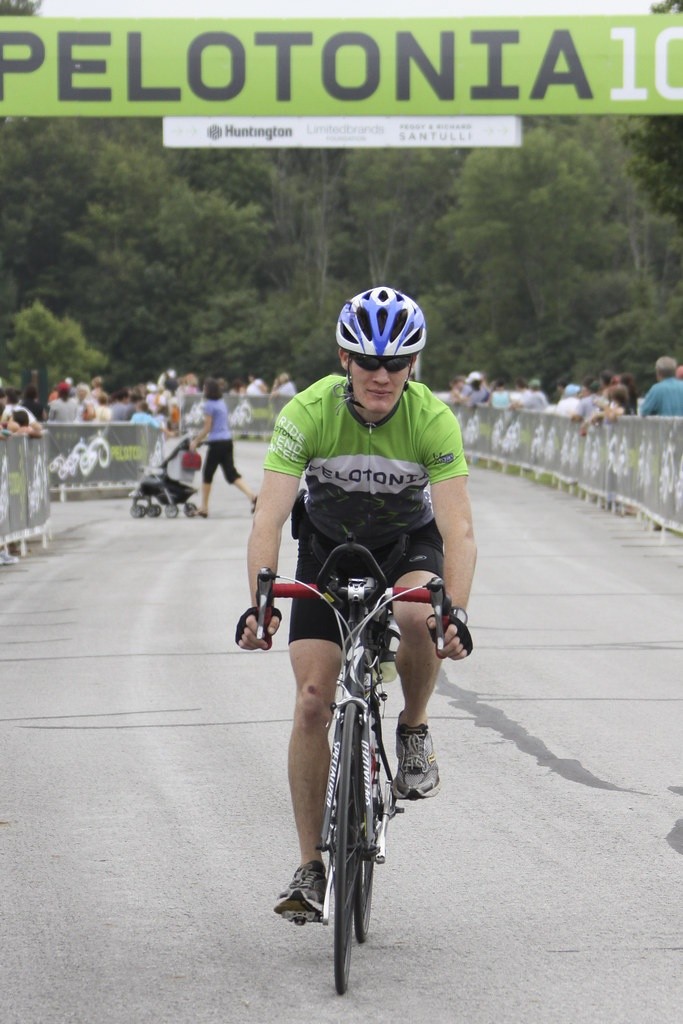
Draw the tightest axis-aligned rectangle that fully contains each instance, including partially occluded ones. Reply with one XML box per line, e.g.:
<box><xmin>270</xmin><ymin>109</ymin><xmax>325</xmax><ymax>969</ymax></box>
<box><xmin>348</xmin><ymin>350</ymin><xmax>412</xmax><ymax>372</ymax></box>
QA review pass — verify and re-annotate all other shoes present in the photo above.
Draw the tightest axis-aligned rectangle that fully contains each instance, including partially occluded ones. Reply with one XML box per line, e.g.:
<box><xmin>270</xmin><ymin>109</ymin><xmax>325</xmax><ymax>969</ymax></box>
<box><xmin>251</xmin><ymin>496</ymin><xmax>258</xmax><ymax>513</ymax></box>
<box><xmin>192</xmin><ymin>510</ymin><xmax>208</xmax><ymax>518</ymax></box>
<box><xmin>0</xmin><ymin>550</ymin><xmax>18</xmax><ymax>565</ymax></box>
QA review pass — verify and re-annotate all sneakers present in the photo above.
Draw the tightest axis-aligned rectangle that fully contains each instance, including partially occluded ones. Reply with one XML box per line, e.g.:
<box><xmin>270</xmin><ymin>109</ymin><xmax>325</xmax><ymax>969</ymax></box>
<box><xmin>392</xmin><ymin>710</ymin><xmax>440</xmax><ymax>799</ymax></box>
<box><xmin>273</xmin><ymin>860</ymin><xmax>327</xmax><ymax>915</ymax></box>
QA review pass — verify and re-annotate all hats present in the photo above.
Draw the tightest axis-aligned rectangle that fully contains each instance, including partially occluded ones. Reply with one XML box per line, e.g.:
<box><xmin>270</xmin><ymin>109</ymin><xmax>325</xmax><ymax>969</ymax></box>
<box><xmin>56</xmin><ymin>383</ymin><xmax>69</xmax><ymax>393</ymax></box>
<box><xmin>464</xmin><ymin>371</ymin><xmax>483</xmax><ymax>383</ymax></box>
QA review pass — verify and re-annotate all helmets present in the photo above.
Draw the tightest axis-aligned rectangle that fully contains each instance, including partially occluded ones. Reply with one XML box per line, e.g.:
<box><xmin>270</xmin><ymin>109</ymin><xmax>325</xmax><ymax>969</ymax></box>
<box><xmin>336</xmin><ymin>286</ymin><xmax>427</xmax><ymax>356</ymax></box>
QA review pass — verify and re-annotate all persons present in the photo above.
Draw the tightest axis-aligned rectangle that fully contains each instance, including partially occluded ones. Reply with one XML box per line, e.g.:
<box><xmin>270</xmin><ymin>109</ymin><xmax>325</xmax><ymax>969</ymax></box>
<box><xmin>235</xmin><ymin>287</ymin><xmax>478</xmax><ymax>920</ymax></box>
<box><xmin>189</xmin><ymin>377</ymin><xmax>258</xmax><ymax>518</ymax></box>
<box><xmin>177</xmin><ymin>371</ymin><xmax>296</xmax><ymax>397</ymax></box>
<box><xmin>0</xmin><ymin>369</ymin><xmax>180</xmax><ymax>444</ymax></box>
<box><xmin>448</xmin><ymin>356</ymin><xmax>683</xmax><ymax>436</ymax></box>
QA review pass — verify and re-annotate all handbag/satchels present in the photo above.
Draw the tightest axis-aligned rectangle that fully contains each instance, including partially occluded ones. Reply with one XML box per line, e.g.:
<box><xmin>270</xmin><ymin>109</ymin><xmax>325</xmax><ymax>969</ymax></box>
<box><xmin>183</xmin><ymin>449</ymin><xmax>201</xmax><ymax>470</ymax></box>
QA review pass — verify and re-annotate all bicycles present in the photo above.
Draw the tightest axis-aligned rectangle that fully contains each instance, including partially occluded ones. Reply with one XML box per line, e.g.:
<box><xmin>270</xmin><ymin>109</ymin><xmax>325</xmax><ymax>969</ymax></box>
<box><xmin>255</xmin><ymin>532</ymin><xmax>453</xmax><ymax>996</ymax></box>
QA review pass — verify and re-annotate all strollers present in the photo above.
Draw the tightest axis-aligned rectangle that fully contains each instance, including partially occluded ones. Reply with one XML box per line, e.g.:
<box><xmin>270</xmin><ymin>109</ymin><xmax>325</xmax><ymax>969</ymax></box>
<box><xmin>128</xmin><ymin>432</ymin><xmax>198</xmax><ymax>518</ymax></box>
